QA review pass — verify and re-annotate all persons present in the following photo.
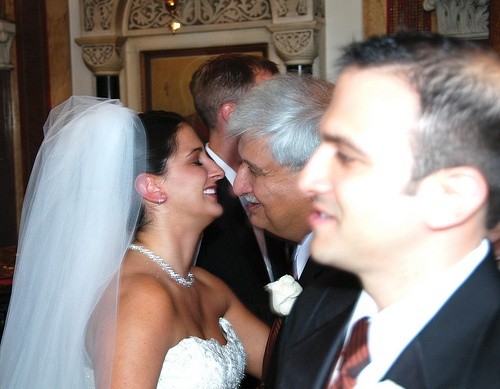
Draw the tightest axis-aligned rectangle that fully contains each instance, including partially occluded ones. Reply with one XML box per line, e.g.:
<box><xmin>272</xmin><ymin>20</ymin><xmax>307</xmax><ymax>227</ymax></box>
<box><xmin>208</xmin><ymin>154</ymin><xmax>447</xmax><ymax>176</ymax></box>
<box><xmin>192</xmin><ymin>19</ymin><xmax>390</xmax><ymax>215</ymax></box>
<box><xmin>176</xmin><ymin>53</ymin><xmax>298</xmax><ymax>389</ymax></box>
<box><xmin>1</xmin><ymin>97</ymin><xmax>274</xmax><ymax>389</ymax></box>
<box><xmin>296</xmin><ymin>27</ymin><xmax>500</xmax><ymax>389</ymax></box>
<box><xmin>219</xmin><ymin>69</ymin><xmax>368</xmax><ymax>389</ymax></box>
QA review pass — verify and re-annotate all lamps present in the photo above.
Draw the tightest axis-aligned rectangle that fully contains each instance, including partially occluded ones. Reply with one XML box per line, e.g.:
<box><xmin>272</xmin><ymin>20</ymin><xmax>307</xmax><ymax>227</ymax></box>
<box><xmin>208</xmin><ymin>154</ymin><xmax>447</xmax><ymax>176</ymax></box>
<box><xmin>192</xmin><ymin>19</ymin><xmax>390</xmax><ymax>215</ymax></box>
<box><xmin>165</xmin><ymin>0</ymin><xmax>181</xmax><ymax>34</ymax></box>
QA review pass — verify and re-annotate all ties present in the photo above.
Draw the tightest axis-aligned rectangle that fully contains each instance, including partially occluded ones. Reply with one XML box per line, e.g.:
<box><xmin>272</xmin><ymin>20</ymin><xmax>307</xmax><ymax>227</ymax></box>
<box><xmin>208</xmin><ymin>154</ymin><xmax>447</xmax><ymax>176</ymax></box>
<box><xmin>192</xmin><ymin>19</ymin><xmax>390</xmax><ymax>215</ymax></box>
<box><xmin>261</xmin><ymin>256</ymin><xmax>298</xmax><ymax>388</ymax></box>
<box><xmin>329</xmin><ymin>318</ymin><xmax>372</xmax><ymax>389</ymax></box>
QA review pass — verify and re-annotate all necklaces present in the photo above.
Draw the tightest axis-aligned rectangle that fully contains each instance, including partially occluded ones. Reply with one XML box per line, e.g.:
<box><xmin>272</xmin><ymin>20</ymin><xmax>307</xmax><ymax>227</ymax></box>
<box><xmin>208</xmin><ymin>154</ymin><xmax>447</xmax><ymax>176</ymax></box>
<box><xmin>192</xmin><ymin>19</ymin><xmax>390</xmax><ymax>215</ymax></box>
<box><xmin>126</xmin><ymin>241</ymin><xmax>197</xmax><ymax>288</ymax></box>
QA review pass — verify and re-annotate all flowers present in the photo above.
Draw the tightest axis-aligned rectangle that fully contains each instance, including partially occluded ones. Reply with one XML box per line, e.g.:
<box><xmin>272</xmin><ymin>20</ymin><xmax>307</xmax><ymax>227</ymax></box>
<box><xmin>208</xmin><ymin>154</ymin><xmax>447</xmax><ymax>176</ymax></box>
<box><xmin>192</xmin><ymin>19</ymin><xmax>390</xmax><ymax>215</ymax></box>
<box><xmin>264</xmin><ymin>274</ymin><xmax>303</xmax><ymax>325</ymax></box>
